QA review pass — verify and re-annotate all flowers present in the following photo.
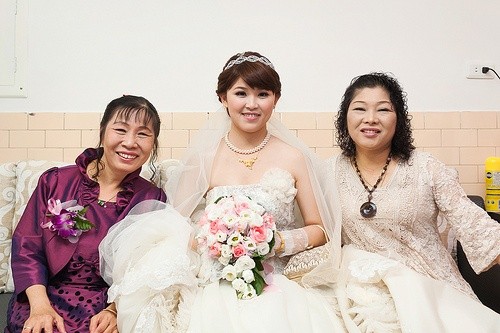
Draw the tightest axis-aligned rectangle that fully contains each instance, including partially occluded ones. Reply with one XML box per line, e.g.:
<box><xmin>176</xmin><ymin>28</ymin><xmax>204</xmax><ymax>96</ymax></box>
<box><xmin>40</xmin><ymin>197</ymin><xmax>95</xmax><ymax>243</ymax></box>
<box><xmin>195</xmin><ymin>194</ymin><xmax>276</xmax><ymax>299</ymax></box>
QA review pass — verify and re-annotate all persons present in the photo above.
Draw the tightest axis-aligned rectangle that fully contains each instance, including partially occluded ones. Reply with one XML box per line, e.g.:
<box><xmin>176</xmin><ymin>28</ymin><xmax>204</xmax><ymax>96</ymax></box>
<box><xmin>326</xmin><ymin>72</ymin><xmax>500</xmax><ymax>333</ymax></box>
<box><xmin>11</xmin><ymin>94</ymin><xmax>167</xmax><ymax>333</ymax></box>
<box><xmin>172</xmin><ymin>51</ymin><xmax>333</xmax><ymax>333</ymax></box>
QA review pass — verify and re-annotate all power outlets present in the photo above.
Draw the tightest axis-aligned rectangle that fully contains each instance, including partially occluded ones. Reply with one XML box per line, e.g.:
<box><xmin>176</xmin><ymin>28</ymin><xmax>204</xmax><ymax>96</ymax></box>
<box><xmin>466</xmin><ymin>64</ymin><xmax>496</xmax><ymax>78</ymax></box>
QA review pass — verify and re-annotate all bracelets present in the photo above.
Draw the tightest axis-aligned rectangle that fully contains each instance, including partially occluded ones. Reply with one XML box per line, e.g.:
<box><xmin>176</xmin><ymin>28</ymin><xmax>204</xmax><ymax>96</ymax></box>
<box><xmin>274</xmin><ymin>231</ymin><xmax>285</xmax><ymax>253</ymax></box>
<box><xmin>102</xmin><ymin>308</ymin><xmax>117</xmax><ymax>315</ymax></box>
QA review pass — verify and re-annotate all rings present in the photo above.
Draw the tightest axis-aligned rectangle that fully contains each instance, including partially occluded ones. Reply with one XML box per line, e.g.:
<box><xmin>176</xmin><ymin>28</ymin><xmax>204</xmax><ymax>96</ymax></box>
<box><xmin>24</xmin><ymin>326</ymin><xmax>32</xmax><ymax>330</ymax></box>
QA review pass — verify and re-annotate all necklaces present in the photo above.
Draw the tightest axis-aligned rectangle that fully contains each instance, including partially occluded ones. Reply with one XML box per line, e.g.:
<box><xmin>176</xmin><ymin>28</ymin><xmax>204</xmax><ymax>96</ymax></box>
<box><xmin>353</xmin><ymin>149</ymin><xmax>393</xmax><ymax>218</ymax></box>
<box><xmin>225</xmin><ymin>130</ymin><xmax>270</xmax><ymax>154</ymax></box>
<box><xmin>235</xmin><ymin>152</ymin><xmax>260</xmax><ymax>170</ymax></box>
<box><xmin>97</xmin><ymin>189</ymin><xmax>122</xmax><ymax>209</ymax></box>
<box><xmin>360</xmin><ymin>170</ymin><xmax>386</xmax><ymax>189</ymax></box>
